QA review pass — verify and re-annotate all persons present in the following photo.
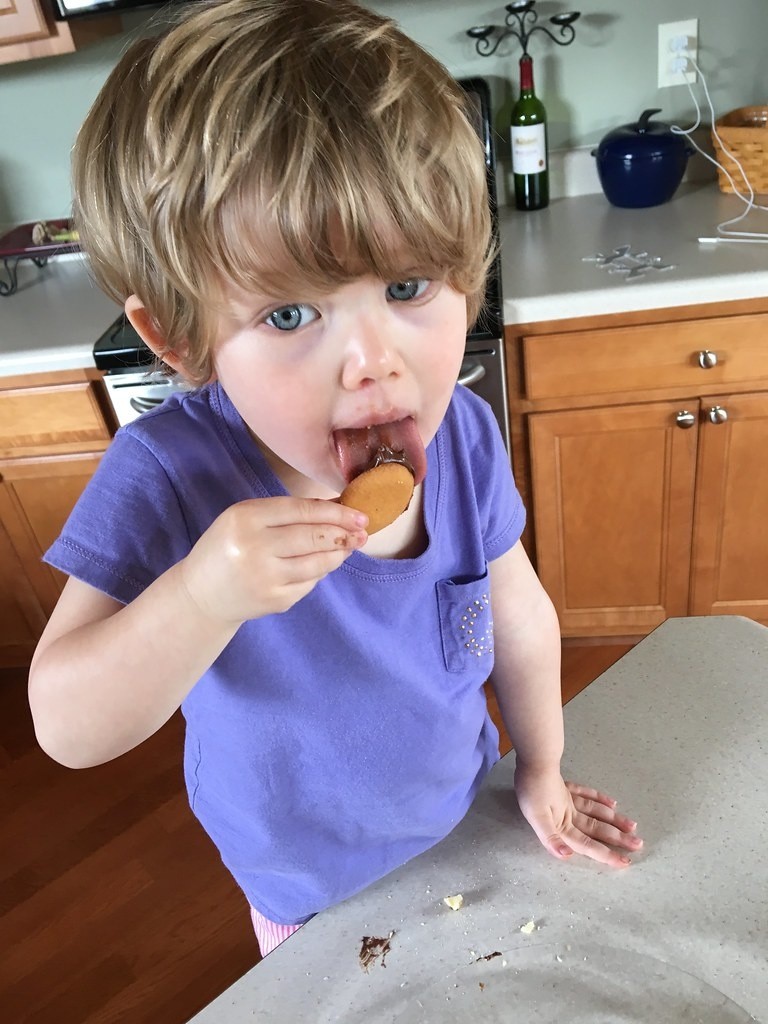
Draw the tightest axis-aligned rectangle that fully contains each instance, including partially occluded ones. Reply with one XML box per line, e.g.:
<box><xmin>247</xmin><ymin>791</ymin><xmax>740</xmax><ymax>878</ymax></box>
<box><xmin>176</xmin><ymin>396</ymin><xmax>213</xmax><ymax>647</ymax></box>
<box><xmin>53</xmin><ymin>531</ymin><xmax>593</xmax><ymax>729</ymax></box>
<box><xmin>29</xmin><ymin>0</ymin><xmax>644</xmax><ymax>957</ymax></box>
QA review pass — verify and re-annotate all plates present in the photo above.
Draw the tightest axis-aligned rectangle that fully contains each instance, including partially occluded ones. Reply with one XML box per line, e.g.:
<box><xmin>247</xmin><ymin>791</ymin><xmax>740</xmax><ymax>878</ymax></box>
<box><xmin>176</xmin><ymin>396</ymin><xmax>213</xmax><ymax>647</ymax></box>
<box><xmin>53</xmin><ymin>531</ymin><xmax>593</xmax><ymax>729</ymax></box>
<box><xmin>0</xmin><ymin>218</ymin><xmax>89</xmax><ymax>260</ymax></box>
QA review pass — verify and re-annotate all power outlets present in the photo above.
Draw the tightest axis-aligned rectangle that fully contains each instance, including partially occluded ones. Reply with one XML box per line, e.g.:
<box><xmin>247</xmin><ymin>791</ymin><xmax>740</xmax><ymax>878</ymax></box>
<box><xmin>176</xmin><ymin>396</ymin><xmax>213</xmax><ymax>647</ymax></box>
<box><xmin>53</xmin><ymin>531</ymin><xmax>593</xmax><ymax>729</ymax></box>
<box><xmin>657</xmin><ymin>17</ymin><xmax>698</xmax><ymax>88</ymax></box>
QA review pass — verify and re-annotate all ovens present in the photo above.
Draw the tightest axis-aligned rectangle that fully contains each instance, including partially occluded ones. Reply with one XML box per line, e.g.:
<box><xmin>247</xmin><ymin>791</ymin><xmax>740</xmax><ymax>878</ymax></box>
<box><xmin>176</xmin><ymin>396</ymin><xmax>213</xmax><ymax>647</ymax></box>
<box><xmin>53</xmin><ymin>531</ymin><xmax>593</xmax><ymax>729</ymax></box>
<box><xmin>99</xmin><ymin>337</ymin><xmax>513</xmax><ymax>470</ymax></box>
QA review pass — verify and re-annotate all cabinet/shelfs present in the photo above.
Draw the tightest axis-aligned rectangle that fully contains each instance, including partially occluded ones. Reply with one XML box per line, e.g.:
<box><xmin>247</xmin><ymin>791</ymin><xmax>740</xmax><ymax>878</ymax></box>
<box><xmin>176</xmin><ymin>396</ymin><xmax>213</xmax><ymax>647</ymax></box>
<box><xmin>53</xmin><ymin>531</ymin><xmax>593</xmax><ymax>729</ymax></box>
<box><xmin>0</xmin><ymin>367</ymin><xmax>121</xmax><ymax>670</ymax></box>
<box><xmin>504</xmin><ymin>297</ymin><xmax>768</xmax><ymax>647</ymax></box>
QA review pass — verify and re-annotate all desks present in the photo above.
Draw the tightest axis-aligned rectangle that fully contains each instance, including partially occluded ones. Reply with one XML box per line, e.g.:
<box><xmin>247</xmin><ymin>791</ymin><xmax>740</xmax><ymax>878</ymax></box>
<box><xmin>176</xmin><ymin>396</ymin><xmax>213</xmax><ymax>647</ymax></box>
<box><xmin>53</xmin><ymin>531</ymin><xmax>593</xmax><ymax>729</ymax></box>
<box><xmin>186</xmin><ymin>615</ymin><xmax>768</xmax><ymax>1024</ymax></box>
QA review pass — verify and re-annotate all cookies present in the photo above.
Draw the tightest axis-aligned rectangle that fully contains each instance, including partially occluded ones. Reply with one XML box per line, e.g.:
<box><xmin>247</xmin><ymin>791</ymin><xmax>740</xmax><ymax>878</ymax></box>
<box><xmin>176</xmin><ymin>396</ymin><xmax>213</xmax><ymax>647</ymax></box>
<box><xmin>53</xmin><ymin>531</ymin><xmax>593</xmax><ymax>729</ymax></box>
<box><xmin>341</xmin><ymin>463</ymin><xmax>415</xmax><ymax>535</ymax></box>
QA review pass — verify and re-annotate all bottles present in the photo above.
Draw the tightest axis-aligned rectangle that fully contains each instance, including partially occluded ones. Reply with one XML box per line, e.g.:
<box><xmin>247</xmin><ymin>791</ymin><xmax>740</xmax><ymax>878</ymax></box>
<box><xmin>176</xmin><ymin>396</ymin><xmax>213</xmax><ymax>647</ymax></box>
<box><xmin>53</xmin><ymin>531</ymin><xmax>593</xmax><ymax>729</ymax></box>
<box><xmin>511</xmin><ymin>59</ymin><xmax>550</xmax><ymax>210</ymax></box>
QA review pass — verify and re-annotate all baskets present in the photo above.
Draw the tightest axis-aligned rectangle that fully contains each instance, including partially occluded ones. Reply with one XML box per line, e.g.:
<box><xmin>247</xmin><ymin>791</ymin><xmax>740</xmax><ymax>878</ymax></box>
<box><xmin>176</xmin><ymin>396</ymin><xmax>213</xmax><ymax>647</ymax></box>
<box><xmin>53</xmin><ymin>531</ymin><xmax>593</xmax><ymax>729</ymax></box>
<box><xmin>711</xmin><ymin>105</ymin><xmax>768</xmax><ymax>195</ymax></box>
